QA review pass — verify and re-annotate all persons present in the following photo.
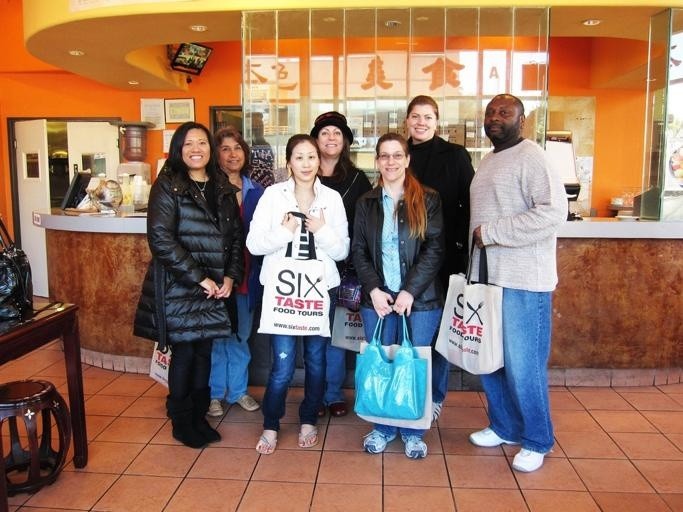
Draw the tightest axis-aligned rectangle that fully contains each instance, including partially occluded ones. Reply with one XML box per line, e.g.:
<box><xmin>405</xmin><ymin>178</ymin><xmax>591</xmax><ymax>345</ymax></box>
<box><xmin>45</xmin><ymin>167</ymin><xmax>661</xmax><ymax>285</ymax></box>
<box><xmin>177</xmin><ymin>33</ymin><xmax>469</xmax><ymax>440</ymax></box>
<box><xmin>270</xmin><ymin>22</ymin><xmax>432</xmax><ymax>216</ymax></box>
<box><xmin>350</xmin><ymin>133</ymin><xmax>446</xmax><ymax>461</ymax></box>
<box><xmin>310</xmin><ymin>113</ymin><xmax>374</xmax><ymax>418</ymax></box>
<box><xmin>132</xmin><ymin>122</ymin><xmax>244</xmax><ymax>448</ymax></box>
<box><xmin>199</xmin><ymin>128</ymin><xmax>265</xmax><ymax>417</ymax></box>
<box><xmin>242</xmin><ymin>134</ymin><xmax>348</xmax><ymax>454</ymax></box>
<box><xmin>461</xmin><ymin>94</ymin><xmax>568</xmax><ymax>473</ymax></box>
<box><xmin>398</xmin><ymin>96</ymin><xmax>475</xmax><ymax>416</ymax></box>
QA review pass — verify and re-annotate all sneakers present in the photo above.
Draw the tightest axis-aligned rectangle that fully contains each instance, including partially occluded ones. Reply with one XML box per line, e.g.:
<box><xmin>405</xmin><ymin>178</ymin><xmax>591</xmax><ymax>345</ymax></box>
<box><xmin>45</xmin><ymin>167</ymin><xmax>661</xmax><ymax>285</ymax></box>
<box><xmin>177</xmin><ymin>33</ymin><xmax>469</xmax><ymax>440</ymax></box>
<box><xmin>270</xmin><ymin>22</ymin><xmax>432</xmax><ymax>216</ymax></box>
<box><xmin>512</xmin><ymin>447</ymin><xmax>556</xmax><ymax>473</ymax></box>
<box><xmin>469</xmin><ymin>427</ymin><xmax>521</xmax><ymax>448</ymax></box>
<box><xmin>364</xmin><ymin>429</ymin><xmax>398</xmax><ymax>454</ymax></box>
<box><xmin>173</xmin><ymin>423</ymin><xmax>208</xmax><ymax>449</ymax></box>
<box><xmin>198</xmin><ymin>418</ymin><xmax>222</xmax><ymax>443</ymax></box>
<box><xmin>401</xmin><ymin>434</ymin><xmax>428</xmax><ymax>458</ymax></box>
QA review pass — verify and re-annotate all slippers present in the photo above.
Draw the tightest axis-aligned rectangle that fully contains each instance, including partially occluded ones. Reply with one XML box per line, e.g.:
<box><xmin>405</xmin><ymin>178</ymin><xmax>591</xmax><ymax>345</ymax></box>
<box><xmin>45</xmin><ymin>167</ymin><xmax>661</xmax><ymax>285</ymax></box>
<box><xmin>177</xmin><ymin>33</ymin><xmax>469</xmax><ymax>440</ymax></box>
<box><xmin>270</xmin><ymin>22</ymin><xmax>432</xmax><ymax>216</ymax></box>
<box><xmin>255</xmin><ymin>432</ymin><xmax>277</xmax><ymax>455</ymax></box>
<box><xmin>298</xmin><ymin>426</ymin><xmax>319</xmax><ymax>448</ymax></box>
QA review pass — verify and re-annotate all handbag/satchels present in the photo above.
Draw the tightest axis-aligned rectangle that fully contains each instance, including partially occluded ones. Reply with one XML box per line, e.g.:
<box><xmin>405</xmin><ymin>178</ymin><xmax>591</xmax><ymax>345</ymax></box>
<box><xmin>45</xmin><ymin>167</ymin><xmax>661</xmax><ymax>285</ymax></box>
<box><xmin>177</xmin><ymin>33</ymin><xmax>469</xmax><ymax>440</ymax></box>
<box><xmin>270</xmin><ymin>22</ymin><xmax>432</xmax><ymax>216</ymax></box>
<box><xmin>258</xmin><ymin>256</ymin><xmax>332</xmax><ymax>337</ymax></box>
<box><xmin>0</xmin><ymin>242</ymin><xmax>34</xmax><ymax>324</ymax></box>
<box><xmin>149</xmin><ymin>340</ymin><xmax>172</xmax><ymax>388</ymax></box>
<box><xmin>434</xmin><ymin>273</ymin><xmax>504</xmax><ymax>375</ymax></box>
<box><xmin>331</xmin><ymin>302</ymin><xmax>365</xmax><ymax>353</ymax></box>
<box><xmin>350</xmin><ymin>339</ymin><xmax>433</xmax><ymax>430</ymax></box>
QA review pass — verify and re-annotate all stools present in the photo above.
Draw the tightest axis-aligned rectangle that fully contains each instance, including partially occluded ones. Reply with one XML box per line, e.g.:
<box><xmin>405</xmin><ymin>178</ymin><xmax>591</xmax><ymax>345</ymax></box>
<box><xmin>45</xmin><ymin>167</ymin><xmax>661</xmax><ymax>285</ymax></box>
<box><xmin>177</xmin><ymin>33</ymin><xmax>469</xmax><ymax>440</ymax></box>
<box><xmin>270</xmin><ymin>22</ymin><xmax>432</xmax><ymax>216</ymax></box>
<box><xmin>0</xmin><ymin>377</ymin><xmax>71</xmax><ymax>495</ymax></box>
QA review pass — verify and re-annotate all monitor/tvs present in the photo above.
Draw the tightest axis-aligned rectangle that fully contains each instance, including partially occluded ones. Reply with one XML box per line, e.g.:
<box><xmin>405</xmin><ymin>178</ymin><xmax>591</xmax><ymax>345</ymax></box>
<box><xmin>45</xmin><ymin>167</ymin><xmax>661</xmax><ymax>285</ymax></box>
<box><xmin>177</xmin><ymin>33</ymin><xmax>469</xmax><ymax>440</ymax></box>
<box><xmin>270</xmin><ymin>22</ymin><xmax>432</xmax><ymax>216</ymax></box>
<box><xmin>172</xmin><ymin>41</ymin><xmax>213</xmax><ymax>76</ymax></box>
<box><xmin>61</xmin><ymin>171</ymin><xmax>91</xmax><ymax>210</ymax></box>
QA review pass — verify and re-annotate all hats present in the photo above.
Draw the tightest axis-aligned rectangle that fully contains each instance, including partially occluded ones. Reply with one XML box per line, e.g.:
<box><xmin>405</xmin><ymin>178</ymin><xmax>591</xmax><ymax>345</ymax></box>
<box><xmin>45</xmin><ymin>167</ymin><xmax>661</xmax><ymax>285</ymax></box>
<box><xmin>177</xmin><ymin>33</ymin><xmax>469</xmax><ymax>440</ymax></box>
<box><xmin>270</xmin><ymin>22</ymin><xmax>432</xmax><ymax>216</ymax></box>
<box><xmin>310</xmin><ymin>110</ymin><xmax>353</xmax><ymax>145</ymax></box>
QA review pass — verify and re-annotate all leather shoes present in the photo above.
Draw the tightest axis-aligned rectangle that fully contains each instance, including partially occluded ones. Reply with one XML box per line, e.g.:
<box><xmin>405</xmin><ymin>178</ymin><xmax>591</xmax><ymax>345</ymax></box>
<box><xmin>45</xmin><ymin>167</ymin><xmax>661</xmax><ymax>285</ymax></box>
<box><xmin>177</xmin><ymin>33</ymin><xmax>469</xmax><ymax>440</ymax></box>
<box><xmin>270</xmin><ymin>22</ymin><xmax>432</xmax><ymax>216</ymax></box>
<box><xmin>318</xmin><ymin>402</ymin><xmax>348</xmax><ymax>418</ymax></box>
<box><xmin>206</xmin><ymin>400</ymin><xmax>224</xmax><ymax>417</ymax></box>
<box><xmin>236</xmin><ymin>394</ymin><xmax>260</xmax><ymax>411</ymax></box>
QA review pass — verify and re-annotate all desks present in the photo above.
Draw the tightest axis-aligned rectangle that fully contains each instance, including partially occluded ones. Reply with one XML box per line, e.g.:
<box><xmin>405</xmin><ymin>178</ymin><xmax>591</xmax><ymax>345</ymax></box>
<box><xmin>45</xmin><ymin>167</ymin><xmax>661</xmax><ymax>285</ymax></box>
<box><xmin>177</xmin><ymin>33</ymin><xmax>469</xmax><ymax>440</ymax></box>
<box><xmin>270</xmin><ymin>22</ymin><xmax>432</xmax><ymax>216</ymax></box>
<box><xmin>0</xmin><ymin>291</ymin><xmax>90</xmax><ymax>512</ymax></box>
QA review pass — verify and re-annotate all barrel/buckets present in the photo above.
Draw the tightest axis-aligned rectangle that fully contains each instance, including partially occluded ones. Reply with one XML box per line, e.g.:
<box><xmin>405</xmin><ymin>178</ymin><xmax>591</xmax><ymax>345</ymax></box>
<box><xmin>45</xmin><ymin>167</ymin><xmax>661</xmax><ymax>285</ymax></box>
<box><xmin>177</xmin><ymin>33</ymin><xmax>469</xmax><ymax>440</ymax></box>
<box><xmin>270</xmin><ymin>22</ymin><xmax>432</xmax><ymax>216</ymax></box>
<box><xmin>124</xmin><ymin>126</ymin><xmax>147</xmax><ymax>161</ymax></box>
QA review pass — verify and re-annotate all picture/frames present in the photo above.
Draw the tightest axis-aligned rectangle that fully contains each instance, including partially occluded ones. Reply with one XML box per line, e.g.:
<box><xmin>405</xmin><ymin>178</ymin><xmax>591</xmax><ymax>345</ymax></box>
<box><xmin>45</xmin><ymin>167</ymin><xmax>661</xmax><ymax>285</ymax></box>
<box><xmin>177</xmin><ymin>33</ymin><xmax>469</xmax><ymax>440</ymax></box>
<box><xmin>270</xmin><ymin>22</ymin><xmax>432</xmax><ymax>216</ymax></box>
<box><xmin>163</xmin><ymin>96</ymin><xmax>196</xmax><ymax>125</ymax></box>
